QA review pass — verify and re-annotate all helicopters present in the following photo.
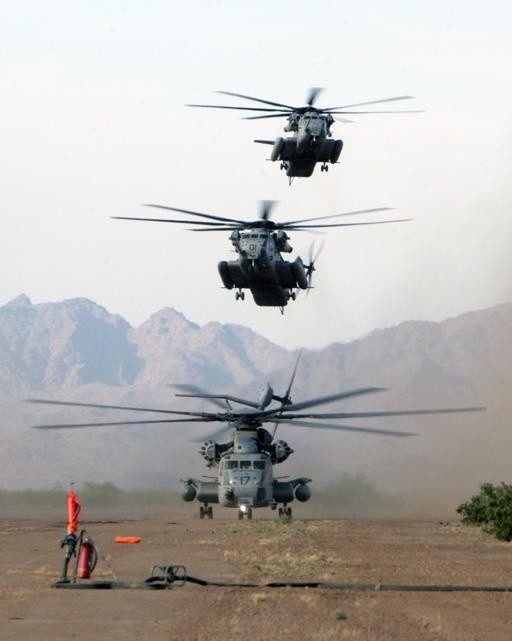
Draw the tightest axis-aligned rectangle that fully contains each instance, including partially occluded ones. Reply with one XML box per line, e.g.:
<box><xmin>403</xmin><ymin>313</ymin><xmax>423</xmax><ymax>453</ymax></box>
<box><xmin>18</xmin><ymin>345</ymin><xmax>488</xmax><ymax>521</ymax></box>
<box><xmin>183</xmin><ymin>86</ymin><xmax>424</xmax><ymax>184</ymax></box>
<box><xmin>106</xmin><ymin>200</ymin><xmax>414</xmax><ymax>315</ymax></box>
